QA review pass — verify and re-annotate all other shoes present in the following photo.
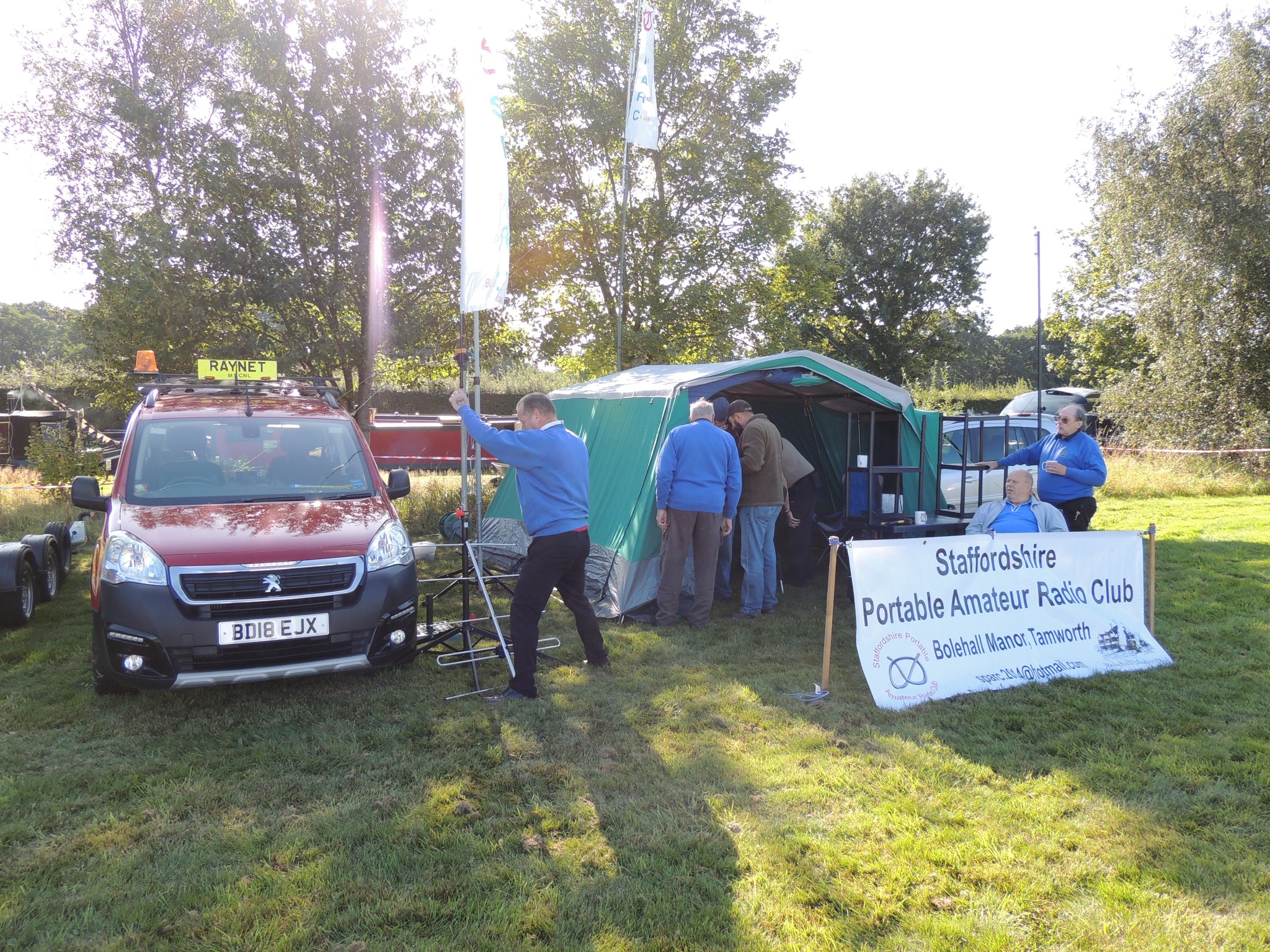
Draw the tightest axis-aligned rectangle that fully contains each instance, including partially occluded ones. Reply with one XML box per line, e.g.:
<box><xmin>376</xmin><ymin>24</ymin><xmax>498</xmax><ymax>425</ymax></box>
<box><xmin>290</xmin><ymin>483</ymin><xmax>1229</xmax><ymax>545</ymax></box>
<box><xmin>591</xmin><ymin>647</ymin><xmax>611</xmax><ymax>667</ymax></box>
<box><xmin>509</xmin><ymin>676</ymin><xmax>538</xmax><ymax>698</ymax></box>
<box><xmin>723</xmin><ymin>606</ymin><xmax>776</xmax><ymax>619</ymax></box>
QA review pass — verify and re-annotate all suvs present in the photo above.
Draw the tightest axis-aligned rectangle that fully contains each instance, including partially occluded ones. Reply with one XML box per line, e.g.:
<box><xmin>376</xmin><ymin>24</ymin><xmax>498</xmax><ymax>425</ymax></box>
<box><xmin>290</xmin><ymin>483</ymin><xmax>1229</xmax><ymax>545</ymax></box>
<box><xmin>71</xmin><ymin>348</ymin><xmax>418</xmax><ymax>695</ymax></box>
<box><xmin>880</xmin><ymin>387</ymin><xmax>1102</xmax><ymax>519</ymax></box>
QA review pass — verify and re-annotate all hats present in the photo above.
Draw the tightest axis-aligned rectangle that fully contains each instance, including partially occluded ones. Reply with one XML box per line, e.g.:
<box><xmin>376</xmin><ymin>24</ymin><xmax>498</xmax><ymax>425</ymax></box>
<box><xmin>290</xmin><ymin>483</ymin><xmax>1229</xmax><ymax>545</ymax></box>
<box><xmin>710</xmin><ymin>397</ymin><xmax>730</xmax><ymax>420</ymax></box>
<box><xmin>727</xmin><ymin>399</ymin><xmax>752</xmax><ymax>416</ymax></box>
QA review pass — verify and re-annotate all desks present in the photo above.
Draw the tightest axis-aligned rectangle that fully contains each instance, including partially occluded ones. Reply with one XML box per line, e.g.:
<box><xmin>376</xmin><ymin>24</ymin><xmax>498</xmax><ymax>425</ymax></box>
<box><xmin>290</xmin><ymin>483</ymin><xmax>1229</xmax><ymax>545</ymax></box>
<box><xmin>847</xmin><ymin>515</ymin><xmax>972</xmax><ymax>541</ymax></box>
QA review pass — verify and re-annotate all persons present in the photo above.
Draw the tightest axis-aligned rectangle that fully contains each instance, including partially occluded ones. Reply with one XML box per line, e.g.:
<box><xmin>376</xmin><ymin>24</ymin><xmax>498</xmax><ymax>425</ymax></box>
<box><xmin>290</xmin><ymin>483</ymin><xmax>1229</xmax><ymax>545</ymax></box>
<box><xmin>709</xmin><ymin>397</ymin><xmax>742</xmax><ymax>604</ymax></box>
<box><xmin>652</xmin><ymin>396</ymin><xmax>742</xmax><ymax>632</ymax></box>
<box><xmin>975</xmin><ymin>405</ymin><xmax>1107</xmax><ymax>531</ymax></box>
<box><xmin>779</xmin><ymin>436</ymin><xmax>817</xmax><ymax>588</ymax></box>
<box><xmin>446</xmin><ymin>389</ymin><xmax>612</xmax><ymax>699</ymax></box>
<box><xmin>727</xmin><ymin>400</ymin><xmax>785</xmax><ymax>622</ymax></box>
<box><xmin>965</xmin><ymin>469</ymin><xmax>1070</xmax><ymax>535</ymax></box>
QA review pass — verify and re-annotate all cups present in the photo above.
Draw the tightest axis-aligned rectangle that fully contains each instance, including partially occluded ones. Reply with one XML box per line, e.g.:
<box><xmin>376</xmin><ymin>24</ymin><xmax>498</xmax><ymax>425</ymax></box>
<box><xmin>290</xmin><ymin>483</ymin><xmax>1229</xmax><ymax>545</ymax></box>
<box><xmin>857</xmin><ymin>455</ymin><xmax>867</xmax><ymax>468</ymax></box>
<box><xmin>915</xmin><ymin>511</ymin><xmax>927</xmax><ymax>525</ymax></box>
<box><xmin>1041</xmin><ymin>460</ymin><xmax>1058</xmax><ymax>472</ymax></box>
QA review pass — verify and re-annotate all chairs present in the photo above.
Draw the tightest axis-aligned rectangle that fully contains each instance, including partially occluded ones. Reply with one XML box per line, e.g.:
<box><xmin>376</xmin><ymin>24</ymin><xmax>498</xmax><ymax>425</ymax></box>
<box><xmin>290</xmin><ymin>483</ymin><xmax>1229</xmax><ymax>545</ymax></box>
<box><xmin>148</xmin><ymin>426</ymin><xmax>225</xmax><ymax>489</ymax></box>
<box><xmin>985</xmin><ymin>434</ymin><xmax>1005</xmax><ymax>461</ymax></box>
<box><xmin>949</xmin><ymin>434</ymin><xmax>963</xmax><ymax>465</ymax></box>
<box><xmin>268</xmin><ymin>431</ymin><xmax>342</xmax><ymax>488</ymax></box>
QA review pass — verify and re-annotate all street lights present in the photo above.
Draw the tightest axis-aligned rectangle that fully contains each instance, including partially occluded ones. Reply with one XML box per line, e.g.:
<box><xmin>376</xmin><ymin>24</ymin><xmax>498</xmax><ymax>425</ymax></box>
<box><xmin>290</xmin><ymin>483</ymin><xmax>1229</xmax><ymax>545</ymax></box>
<box><xmin>1034</xmin><ymin>232</ymin><xmax>1042</xmax><ymax>440</ymax></box>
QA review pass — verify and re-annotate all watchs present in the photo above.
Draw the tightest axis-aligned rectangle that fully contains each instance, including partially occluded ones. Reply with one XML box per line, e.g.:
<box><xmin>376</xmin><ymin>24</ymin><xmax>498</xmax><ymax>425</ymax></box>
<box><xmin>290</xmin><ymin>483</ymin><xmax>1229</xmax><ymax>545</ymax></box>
<box><xmin>784</xmin><ymin>509</ymin><xmax>791</xmax><ymax>514</ymax></box>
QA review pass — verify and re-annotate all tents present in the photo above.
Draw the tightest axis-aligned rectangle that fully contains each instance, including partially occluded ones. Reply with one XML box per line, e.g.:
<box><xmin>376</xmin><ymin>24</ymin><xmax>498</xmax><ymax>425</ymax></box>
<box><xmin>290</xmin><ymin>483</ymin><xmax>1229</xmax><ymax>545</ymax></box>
<box><xmin>472</xmin><ymin>349</ymin><xmax>949</xmax><ymax>634</ymax></box>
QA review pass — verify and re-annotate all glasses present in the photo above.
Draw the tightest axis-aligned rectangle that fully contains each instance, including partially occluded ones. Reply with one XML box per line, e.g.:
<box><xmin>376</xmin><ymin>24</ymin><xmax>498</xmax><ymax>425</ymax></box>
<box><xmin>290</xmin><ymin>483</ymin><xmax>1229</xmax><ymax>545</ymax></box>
<box><xmin>1054</xmin><ymin>416</ymin><xmax>1080</xmax><ymax>424</ymax></box>
<box><xmin>714</xmin><ymin>420</ymin><xmax>723</xmax><ymax>426</ymax></box>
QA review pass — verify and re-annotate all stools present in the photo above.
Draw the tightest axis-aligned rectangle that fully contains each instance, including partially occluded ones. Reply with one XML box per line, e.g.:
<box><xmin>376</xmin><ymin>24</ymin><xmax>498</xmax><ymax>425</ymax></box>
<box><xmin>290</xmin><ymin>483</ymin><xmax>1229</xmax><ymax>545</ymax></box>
<box><xmin>936</xmin><ymin>412</ymin><xmax>1009</xmax><ymax>519</ymax></box>
<box><xmin>845</xmin><ymin>410</ymin><xmax>926</xmax><ymax>524</ymax></box>
